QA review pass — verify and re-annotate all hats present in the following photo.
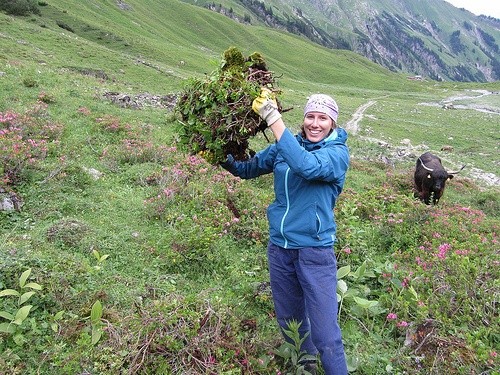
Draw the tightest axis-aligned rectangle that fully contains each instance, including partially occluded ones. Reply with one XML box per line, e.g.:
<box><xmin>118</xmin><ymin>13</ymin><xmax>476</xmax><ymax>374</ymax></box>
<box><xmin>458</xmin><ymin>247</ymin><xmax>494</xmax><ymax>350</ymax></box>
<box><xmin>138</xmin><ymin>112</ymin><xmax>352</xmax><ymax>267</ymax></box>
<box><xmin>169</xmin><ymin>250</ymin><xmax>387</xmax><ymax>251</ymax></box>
<box><xmin>303</xmin><ymin>93</ymin><xmax>339</xmax><ymax>123</ymax></box>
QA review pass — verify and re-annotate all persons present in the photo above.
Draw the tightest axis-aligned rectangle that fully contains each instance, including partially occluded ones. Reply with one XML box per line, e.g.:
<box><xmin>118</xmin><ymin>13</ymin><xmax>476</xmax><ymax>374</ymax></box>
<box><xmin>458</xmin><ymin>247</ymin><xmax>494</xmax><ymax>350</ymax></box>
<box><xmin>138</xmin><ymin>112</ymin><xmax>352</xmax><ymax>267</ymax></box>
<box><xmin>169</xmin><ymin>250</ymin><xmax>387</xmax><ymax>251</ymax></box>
<box><xmin>218</xmin><ymin>87</ymin><xmax>348</xmax><ymax>375</ymax></box>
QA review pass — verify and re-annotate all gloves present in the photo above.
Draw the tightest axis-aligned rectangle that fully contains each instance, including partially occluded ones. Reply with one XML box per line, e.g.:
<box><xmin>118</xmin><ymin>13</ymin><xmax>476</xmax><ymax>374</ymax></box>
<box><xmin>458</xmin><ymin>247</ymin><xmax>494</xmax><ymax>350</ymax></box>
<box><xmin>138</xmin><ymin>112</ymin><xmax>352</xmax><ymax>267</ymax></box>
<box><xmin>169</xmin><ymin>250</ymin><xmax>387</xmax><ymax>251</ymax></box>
<box><xmin>252</xmin><ymin>88</ymin><xmax>284</xmax><ymax>125</ymax></box>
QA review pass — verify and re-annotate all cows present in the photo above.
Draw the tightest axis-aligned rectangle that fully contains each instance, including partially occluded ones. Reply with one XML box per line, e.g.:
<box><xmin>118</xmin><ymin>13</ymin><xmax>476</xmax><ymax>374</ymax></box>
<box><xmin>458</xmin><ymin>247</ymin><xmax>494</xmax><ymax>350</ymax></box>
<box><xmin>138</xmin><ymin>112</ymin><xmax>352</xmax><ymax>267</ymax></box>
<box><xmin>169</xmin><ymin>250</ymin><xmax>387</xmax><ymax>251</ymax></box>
<box><xmin>414</xmin><ymin>152</ymin><xmax>466</xmax><ymax>205</ymax></box>
